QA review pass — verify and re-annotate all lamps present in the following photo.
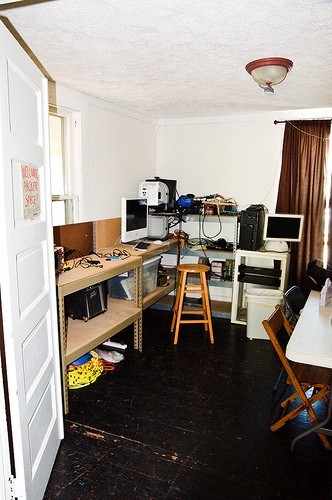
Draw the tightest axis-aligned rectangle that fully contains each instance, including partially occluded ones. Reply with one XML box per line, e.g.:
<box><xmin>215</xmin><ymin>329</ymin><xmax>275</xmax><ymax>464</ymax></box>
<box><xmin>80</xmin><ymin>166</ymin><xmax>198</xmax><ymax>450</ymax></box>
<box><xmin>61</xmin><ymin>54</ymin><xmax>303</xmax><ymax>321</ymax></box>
<box><xmin>246</xmin><ymin>58</ymin><xmax>293</xmax><ymax>89</ymax></box>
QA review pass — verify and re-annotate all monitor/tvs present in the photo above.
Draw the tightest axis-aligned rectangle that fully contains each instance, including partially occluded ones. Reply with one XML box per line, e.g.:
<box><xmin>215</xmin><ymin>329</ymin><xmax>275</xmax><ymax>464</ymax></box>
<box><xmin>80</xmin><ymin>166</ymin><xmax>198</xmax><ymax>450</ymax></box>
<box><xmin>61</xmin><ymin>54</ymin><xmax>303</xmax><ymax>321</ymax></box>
<box><xmin>262</xmin><ymin>214</ymin><xmax>304</xmax><ymax>252</ymax></box>
<box><xmin>121</xmin><ymin>197</ymin><xmax>148</xmax><ymax>242</ymax></box>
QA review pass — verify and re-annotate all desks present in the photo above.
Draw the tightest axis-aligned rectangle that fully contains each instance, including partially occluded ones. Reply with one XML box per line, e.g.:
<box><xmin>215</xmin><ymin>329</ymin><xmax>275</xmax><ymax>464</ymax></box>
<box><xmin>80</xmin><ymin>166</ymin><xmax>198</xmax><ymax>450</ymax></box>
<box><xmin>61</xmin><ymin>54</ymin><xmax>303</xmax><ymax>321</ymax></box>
<box><xmin>284</xmin><ymin>289</ymin><xmax>332</xmax><ymax>451</ymax></box>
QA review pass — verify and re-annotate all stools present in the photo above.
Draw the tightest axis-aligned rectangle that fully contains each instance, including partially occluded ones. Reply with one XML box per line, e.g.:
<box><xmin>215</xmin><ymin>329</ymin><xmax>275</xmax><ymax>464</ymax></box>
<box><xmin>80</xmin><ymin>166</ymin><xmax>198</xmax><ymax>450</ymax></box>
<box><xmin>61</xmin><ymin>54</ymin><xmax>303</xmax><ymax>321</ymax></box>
<box><xmin>169</xmin><ymin>264</ymin><xmax>215</xmax><ymax>345</ymax></box>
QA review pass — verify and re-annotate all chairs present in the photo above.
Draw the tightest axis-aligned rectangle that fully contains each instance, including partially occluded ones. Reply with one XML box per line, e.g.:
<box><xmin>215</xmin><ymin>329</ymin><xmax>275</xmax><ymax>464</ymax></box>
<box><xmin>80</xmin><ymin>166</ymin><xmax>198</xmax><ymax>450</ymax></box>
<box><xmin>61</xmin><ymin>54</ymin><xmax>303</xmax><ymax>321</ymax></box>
<box><xmin>262</xmin><ymin>286</ymin><xmax>332</xmax><ymax>451</ymax></box>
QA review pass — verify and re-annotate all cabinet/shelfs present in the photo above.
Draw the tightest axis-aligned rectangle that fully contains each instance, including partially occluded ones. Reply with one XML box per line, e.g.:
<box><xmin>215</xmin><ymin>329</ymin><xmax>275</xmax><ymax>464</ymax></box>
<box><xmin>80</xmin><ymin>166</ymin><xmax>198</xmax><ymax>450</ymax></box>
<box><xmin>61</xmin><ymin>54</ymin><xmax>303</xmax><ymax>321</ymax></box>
<box><xmin>147</xmin><ymin>214</ymin><xmax>240</xmax><ymax>319</ymax></box>
<box><xmin>230</xmin><ymin>247</ymin><xmax>291</xmax><ymax>325</ymax></box>
<box><xmin>101</xmin><ymin>236</ymin><xmax>181</xmax><ymax>349</ymax></box>
<box><xmin>56</xmin><ymin>253</ymin><xmax>143</xmax><ymax>414</ymax></box>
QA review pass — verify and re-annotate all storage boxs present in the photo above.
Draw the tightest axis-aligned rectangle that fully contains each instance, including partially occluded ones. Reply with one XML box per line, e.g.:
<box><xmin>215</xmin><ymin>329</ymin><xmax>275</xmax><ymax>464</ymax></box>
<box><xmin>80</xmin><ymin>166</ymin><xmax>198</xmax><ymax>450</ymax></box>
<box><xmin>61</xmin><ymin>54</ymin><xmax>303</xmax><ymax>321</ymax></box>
<box><xmin>106</xmin><ymin>254</ymin><xmax>162</xmax><ymax>301</ymax></box>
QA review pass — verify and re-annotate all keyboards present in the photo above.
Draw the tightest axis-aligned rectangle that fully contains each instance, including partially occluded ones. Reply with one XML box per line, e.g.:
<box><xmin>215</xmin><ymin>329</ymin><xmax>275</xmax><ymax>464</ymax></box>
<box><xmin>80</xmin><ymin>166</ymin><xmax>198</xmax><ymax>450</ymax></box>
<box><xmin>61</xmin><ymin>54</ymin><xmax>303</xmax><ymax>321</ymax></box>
<box><xmin>133</xmin><ymin>242</ymin><xmax>151</xmax><ymax>251</ymax></box>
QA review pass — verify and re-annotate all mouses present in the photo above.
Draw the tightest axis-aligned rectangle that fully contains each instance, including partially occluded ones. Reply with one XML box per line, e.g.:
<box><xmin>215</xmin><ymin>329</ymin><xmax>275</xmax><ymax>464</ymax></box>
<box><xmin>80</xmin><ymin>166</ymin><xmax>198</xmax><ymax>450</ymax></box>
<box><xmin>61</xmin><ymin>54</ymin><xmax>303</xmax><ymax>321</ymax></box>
<box><xmin>152</xmin><ymin>240</ymin><xmax>163</xmax><ymax>244</ymax></box>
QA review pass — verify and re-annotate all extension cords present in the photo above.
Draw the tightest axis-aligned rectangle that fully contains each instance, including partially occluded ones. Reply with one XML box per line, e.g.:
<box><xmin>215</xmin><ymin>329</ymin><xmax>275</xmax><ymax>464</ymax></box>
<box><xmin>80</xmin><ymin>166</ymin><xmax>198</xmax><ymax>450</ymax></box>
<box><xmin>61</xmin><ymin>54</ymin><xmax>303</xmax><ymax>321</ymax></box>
<box><xmin>103</xmin><ymin>341</ymin><xmax>127</xmax><ymax>349</ymax></box>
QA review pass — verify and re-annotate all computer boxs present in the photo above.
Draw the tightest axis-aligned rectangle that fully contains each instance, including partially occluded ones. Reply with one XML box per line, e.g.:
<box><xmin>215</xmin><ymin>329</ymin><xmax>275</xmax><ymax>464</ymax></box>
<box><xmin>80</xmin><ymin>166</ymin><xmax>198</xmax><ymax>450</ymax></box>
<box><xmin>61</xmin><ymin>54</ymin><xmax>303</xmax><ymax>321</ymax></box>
<box><xmin>238</xmin><ymin>204</ymin><xmax>265</xmax><ymax>251</ymax></box>
